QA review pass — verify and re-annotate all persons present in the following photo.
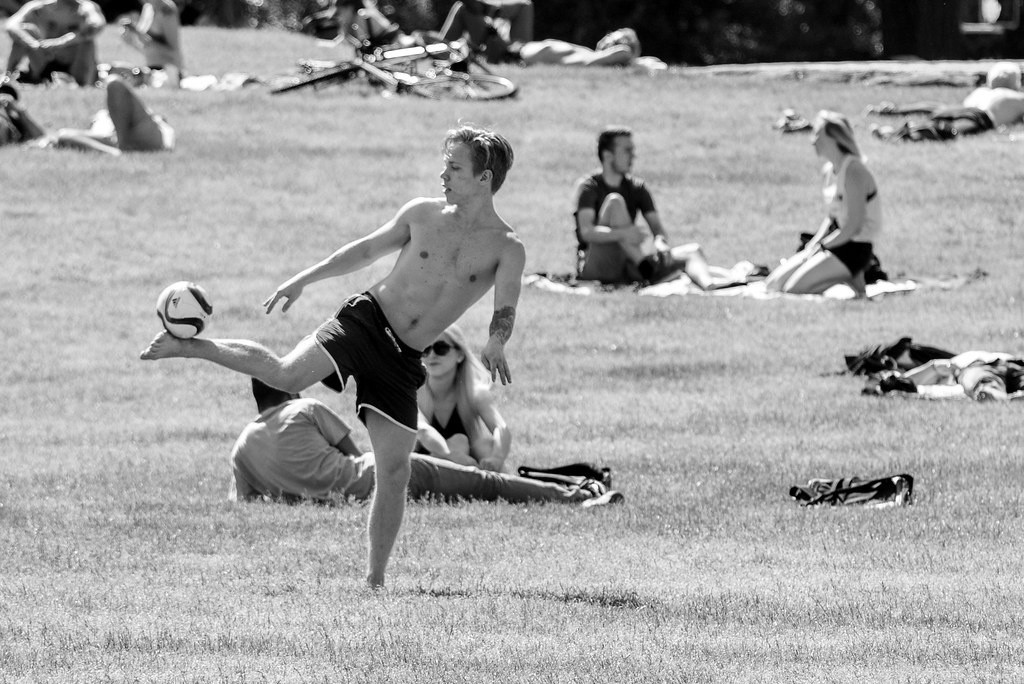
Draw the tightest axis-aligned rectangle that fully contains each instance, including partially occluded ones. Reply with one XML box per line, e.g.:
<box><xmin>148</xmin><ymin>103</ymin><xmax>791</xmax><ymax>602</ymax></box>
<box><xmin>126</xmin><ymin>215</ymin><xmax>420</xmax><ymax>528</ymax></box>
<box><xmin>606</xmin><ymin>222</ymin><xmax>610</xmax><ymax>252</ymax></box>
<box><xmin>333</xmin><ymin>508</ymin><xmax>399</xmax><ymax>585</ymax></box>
<box><xmin>105</xmin><ymin>0</ymin><xmax>191</xmax><ymax>89</ymax></box>
<box><xmin>300</xmin><ymin>0</ymin><xmax>512</xmax><ymax>66</ymax></box>
<box><xmin>863</xmin><ymin>60</ymin><xmax>1024</xmax><ymax>142</ymax></box>
<box><xmin>411</xmin><ymin>326</ymin><xmax>514</xmax><ymax>475</ymax></box>
<box><xmin>421</xmin><ymin>0</ymin><xmax>642</xmax><ymax>66</ymax></box>
<box><xmin>0</xmin><ymin>75</ymin><xmax>48</xmax><ymax>149</ymax></box>
<box><xmin>39</xmin><ymin>76</ymin><xmax>179</xmax><ymax>159</ymax></box>
<box><xmin>571</xmin><ymin>125</ymin><xmax>746</xmax><ymax>292</ymax></box>
<box><xmin>139</xmin><ymin>124</ymin><xmax>530</xmax><ymax>590</ymax></box>
<box><xmin>6</xmin><ymin>0</ymin><xmax>109</xmax><ymax>86</ymax></box>
<box><xmin>766</xmin><ymin>111</ymin><xmax>885</xmax><ymax>300</ymax></box>
<box><xmin>227</xmin><ymin>375</ymin><xmax>627</xmax><ymax>511</ymax></box>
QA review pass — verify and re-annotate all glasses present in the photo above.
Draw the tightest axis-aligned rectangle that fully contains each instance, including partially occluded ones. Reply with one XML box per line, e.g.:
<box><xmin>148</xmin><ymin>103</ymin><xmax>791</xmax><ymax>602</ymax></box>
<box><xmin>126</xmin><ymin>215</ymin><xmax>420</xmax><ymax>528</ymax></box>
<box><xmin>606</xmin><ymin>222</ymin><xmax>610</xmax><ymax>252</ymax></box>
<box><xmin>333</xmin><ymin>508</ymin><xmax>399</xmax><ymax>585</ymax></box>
<box><xmin>421</xmin><ymin>340</ymin><xmax>455</xmax><ymax>358</ymax></box>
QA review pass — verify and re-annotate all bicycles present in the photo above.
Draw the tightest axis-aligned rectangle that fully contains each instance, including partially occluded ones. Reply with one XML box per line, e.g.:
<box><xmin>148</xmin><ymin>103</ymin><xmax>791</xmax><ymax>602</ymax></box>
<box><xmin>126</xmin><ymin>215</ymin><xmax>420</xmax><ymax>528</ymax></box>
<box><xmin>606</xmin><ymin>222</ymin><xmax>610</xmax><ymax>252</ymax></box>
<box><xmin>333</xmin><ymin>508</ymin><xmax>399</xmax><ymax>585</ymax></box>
<box><xmin>296</xmin><ymin>8</ymin><xmax>520</xmax><ymax>101</ymax></box>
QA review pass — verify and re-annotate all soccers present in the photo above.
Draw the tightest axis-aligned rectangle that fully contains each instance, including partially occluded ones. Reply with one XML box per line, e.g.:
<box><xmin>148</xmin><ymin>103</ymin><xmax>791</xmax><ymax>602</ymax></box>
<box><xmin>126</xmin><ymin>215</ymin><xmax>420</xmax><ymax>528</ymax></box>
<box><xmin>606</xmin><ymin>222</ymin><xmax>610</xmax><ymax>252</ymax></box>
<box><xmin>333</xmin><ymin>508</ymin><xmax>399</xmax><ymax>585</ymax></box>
<box><xmin>150</xmin><ymin>279</ymin><xmax>217</xmax><ymax>341</ymax></box>
